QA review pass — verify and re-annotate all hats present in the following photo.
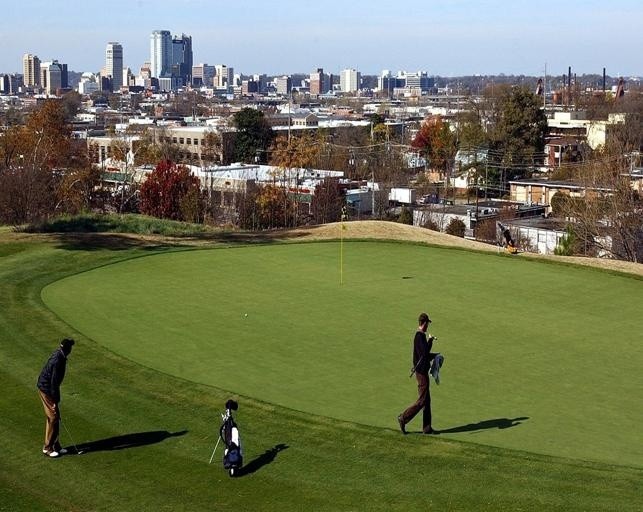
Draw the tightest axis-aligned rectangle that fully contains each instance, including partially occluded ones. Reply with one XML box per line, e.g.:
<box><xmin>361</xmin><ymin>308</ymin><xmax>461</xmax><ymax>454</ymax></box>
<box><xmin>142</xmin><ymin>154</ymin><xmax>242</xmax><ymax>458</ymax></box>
<box><xmin>418</xmin><ymin>313</ymin><xmax>432</xmax><ymax>323</ymax></box>
<box><xmin>59</xmin><ymin>339</ymin><xmax>75</xmax><ymax>348</ymax></box>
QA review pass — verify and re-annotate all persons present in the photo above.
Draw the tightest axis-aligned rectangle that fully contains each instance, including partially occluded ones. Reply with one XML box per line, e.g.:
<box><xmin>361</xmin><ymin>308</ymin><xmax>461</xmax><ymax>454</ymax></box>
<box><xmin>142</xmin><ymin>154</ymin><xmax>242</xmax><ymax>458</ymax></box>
<box><xmin>37</xmin><ymin>338</ymin><xmax>75</xmax><ymax>457</ymax></box>
<box><xmin>397</xmin><ymin>313</ymin><xmax>440</xmax><ymax>434</ymax></box>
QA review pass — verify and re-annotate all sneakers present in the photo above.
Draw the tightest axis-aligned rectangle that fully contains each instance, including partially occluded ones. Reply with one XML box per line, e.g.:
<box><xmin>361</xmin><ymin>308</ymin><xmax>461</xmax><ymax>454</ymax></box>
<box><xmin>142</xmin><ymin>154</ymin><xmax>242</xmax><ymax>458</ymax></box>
<box><xmin>423</xmin><ymin>429</ymin><xmax>440</xmax><ymax>435</ymax></box>
<box><xmin>59</xmin><ymin>448</ymin><xmax>67</xmax><ymax>453</ymax></box>
<box><xmin>398</xmin><ymin>415</ymin><xmax>407</xmax><ymax>434</ymax></box>
<box><xmin>42</xmin><ymin>449</ymin><xmax>59</xmax><ymax>457</ymax></box>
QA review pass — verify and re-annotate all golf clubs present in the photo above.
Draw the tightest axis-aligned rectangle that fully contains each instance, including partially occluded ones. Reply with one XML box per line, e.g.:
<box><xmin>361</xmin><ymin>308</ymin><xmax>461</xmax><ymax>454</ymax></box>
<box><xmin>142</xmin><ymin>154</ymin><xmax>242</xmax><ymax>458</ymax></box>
<box><xmin>409</xmin><ymin>335</ymin><xmax>438</xmax><ymax>379</ymax></box>
<box><xmin>59</xmin><ymin>417</ymin><xmax>84</xmax><ymax>455</ymax></box>
<box><xmin>221</xmin><ymin>400</ymin><xmax>238</xmax><ymax>424</ymax></box>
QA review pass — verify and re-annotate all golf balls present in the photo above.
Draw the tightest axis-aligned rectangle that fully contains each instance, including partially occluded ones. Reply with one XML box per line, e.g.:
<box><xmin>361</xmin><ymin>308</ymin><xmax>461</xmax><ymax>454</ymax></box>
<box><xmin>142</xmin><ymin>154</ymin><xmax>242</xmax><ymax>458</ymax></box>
<box><xmin>245</xmin><ymin>313</ymin><xmax>249</xmax><ymax>317</ymax></box>
